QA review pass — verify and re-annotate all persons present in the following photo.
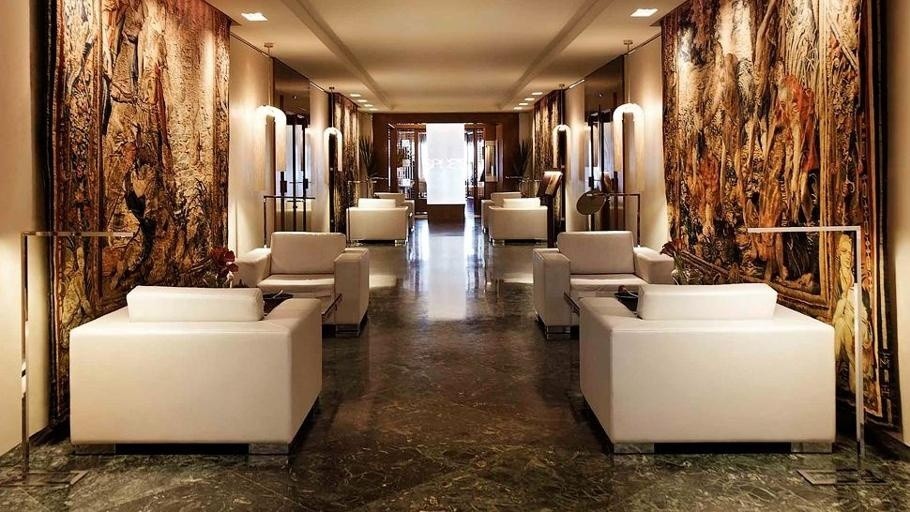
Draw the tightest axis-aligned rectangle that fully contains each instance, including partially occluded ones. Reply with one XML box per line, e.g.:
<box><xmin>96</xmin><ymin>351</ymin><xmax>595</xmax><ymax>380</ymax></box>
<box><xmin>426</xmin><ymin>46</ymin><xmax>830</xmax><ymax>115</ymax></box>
<box><xmin>829</xmin><ymin>232</ymin><xmax>876</xmax><ymax>405</ymax></box>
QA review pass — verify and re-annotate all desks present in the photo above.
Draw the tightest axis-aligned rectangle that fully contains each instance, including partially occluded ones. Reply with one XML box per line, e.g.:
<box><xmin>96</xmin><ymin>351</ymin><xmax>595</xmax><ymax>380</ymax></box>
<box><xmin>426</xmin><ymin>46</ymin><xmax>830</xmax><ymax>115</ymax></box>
<box><xmin>263</xmin><ymin>292</ymin><xmax>343</xmax><ymax>322</ymax></box>
<box><xmin>561</xmin><ymin>288</ymin><xmax>637</xmax><ymax>317</ymax></box>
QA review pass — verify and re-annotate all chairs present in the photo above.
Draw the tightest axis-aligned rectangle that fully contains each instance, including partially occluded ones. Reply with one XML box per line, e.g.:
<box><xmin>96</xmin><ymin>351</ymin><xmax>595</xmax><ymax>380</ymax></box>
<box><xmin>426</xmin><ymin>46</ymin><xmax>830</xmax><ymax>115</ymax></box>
<box><xmin>374</xmin><ymin>191</ymin><xmax>414</xmax><ymax>231</ymax></box>
<box><xmin>480</xmin><ymin>192</ymin><xmax>521</xmax><ymax>233</ymax></box>
<box><xmin>487</xmin><ymin>198</ymin><xmax>547</xmax><ymax>246</ymax></box>
<box><xmin>532</xmin><ymin>230</ymin><xmax>675</xmax><ymax>341</ymax></box>
<box><xmin>579</xmin><ymin>283</ymin><xmax>839</xmax><ymax>454</ymax></box>
<box><xmin>231</xmin><ymin>231</ymin><xmax>369</xmax><ymax>335</ymax></box>
<box><xmin>346</xmin><ymin>198</ymin><xmax>409</xmax><ymax>247</ymax></box>
<box><xmin>67</xmin><ymin>285</ymin><xmax>323</xmax><ymax>453</ymax></box>
